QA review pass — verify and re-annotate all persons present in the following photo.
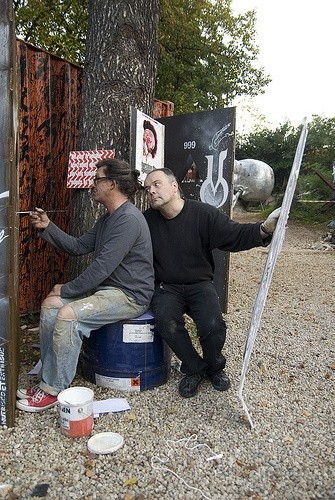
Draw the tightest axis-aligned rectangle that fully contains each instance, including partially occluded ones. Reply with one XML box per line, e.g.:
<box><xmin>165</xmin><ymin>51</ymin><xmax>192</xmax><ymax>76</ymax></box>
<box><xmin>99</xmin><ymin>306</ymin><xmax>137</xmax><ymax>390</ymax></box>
<box><xmin>142</xmin><ymin>168</ymin><xmax>281</xmax><ymax>397</ymax></box>
<box><xmin>16</xmin><ymin>158</ymin><xmax>154</xmax><ymax>412</ymax></box>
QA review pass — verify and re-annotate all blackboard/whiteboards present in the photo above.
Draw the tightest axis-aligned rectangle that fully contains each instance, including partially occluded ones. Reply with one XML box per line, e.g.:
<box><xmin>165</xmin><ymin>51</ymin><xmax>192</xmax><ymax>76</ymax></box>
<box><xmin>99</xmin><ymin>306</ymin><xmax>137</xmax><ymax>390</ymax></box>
<box><xmin>153</xmin><ymin>107</ymin><xmax>237</xmax><ymax>312</ymax></box>
<box><xmin>130</xmin><ymin>108</ymin><xmax>166</xmax><ymax>188</ymax></box>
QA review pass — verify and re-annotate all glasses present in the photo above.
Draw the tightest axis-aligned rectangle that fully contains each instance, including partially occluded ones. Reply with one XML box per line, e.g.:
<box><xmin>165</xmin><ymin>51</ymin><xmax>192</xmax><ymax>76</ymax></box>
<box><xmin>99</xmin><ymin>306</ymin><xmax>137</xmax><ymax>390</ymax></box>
<box><xmin>93</xmin><ymin>176</ymin><xmax>109</xmax><ymax>184</ymax></box>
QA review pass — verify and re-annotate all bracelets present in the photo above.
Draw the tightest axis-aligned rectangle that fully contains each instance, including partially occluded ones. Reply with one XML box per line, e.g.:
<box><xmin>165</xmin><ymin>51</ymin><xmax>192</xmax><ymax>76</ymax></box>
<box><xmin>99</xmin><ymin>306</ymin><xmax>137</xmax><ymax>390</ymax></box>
<box><xmin>260</xmin><ymin>224</ymin><xmax>272</xmax><ymax>236</ymax></box>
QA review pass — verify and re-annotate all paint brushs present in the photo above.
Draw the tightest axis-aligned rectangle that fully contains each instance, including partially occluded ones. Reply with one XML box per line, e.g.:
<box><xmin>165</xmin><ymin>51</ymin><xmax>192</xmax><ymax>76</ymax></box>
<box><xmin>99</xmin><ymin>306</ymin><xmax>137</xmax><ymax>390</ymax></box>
<box><xmin>15</xmin><ymin>210</ymin><xmax>70</xmax><ymax>214</ymax></box>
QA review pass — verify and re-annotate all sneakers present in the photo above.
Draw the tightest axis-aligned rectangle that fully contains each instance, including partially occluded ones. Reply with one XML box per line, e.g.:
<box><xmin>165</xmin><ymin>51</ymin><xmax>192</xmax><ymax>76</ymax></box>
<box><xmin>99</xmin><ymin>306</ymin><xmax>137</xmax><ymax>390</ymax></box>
<box><xmin>16</xmin><ymin>390</ymin><xmax>59</xmax><ymax>411</ymax></box>
<box><xmin>15</xmin><ymin>384</ymin><xmax>40</xmax><ymax>398</ymax></box>
<box><xmin>177</xmin><ymin>370</ymin><xmax>207</xmax><ymax>397</ymax></box>
<box><xmin>208</xmin><ymin>368</ymin><xmax>230</xmax><ymax>392</ymax></box>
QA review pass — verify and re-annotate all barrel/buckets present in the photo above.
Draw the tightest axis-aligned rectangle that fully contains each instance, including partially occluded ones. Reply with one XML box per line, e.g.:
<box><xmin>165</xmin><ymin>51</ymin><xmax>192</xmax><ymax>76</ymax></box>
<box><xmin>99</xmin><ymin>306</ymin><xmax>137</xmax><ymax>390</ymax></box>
<box><xmin>57</xmin><ymin>386</ymin><xmax>94</xmax><ymax>438</ymax></box>
<box><xmin>78</xmin><ymin>310</ymin><xmax>172</xmax><ymax>391</ymax></box>
